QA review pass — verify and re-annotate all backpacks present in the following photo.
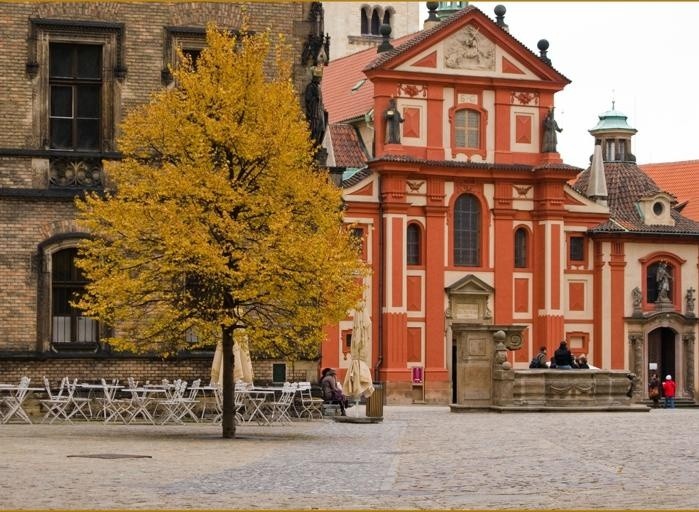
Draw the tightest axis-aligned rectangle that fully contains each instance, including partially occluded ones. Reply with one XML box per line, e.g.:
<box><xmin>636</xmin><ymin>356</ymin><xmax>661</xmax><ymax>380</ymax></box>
<box><xmin>529</xmin><ymin>357</ymin><xmax>541</xmax><ymax>368</ymax></box>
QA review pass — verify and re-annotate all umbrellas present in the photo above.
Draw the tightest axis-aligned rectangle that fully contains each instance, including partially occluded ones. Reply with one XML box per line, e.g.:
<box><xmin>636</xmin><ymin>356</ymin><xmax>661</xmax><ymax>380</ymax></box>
<box><xmin>210</xmin><ymin>308</ymin><xmax>254</xmax><ymax>387</ymax></box>
<box><xmin>342</xmin><ymin>298</ymin><xmax>375</xmax><ymax>403</ymax></box>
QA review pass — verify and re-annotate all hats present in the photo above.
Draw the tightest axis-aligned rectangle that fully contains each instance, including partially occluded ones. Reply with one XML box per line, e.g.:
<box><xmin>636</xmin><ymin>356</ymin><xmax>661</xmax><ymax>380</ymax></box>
<box><xmin>666</xmin><ymin>374</ymin><xmax>672</xmax><ymax>379</ymax></box>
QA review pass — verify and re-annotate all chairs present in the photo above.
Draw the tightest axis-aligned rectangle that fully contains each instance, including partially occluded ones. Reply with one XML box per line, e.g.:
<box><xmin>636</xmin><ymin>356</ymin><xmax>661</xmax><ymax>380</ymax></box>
<box><xmin>0</xmin><ymin>375</ymin><xmax>326</xmax><ymax>424</ymax></box>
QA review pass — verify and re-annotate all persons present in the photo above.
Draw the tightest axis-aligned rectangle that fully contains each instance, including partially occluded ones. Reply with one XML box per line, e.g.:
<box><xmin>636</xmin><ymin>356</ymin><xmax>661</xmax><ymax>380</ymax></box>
<box><xmin>648</xmin><ymin>371</ymin><xmax>677</xmax><ymax>403</ymax></box>
<box><xmin>317</xmin><ymin>363</ymin><xmax>350</xmax><ymax>416</ymax></box>
<box><xmin>382</xmin><ymin>100</ymin><xmax>405</xmax><ymax>145</ymax></box>
<box><xmin>305</xmin><ymin>67</ymin><xmax>329</xmax><ymax>145</ymax></box>
<box><xmin>543</xmin><ymin>111</ymin><xmax>563</xmax><ymax>152</ymax></box>
<box><xmin>657</xmin><ymin>263</ymin><xmax>674</xmax><ymax>306</ymax></box>
<box><xmin>535</xmin><ymin>339</ymin><xmax>592</xmax><ymax>371</ymax></box>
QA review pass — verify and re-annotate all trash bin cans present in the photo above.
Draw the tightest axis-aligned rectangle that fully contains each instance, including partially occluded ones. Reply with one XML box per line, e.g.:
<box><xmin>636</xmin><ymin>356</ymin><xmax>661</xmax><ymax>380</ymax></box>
<box><xmin>365</xmin><ymin>382</ymin><xmax>384</xmax><ymax>418</ymax></box>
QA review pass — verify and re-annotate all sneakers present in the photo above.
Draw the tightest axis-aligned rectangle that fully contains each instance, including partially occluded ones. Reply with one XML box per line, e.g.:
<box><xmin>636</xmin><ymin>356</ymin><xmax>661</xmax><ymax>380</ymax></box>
<box><xmin>341</xmin><ymin>403</ymin><xmax>353</xmax><ymax>416</ymax></box>
